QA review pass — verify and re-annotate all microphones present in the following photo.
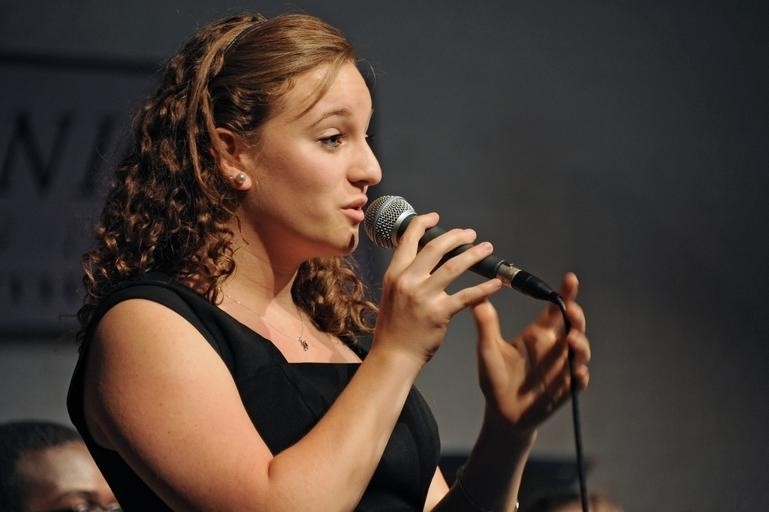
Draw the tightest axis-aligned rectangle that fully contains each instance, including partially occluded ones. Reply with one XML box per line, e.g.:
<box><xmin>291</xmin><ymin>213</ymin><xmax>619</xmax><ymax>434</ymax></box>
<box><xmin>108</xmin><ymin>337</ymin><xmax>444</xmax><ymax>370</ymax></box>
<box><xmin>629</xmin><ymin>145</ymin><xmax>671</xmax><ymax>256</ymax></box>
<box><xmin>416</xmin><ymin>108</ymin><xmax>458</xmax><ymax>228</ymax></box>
<box><xmin>363</xmin><ymin>196</ymin><xmax>558</xmax><ymax>304</ymax></box>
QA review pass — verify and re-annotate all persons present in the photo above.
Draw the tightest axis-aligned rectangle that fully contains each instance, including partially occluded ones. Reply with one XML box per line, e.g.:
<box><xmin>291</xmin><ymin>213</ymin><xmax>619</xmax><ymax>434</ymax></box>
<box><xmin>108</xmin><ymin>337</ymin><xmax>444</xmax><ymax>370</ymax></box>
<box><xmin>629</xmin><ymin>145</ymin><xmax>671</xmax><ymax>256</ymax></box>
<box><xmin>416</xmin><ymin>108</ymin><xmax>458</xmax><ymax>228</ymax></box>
<box><xmin>441</xmin><ymin>452</ymin><xmax>616</xmax><ymax>512</ymax></box>
<box><xmin>63</xmin><ymin>9</ymin><xmax>594</xmax><ymax>512</ymax></box>
<box><xmin>0</xmin><ymin>419</ymin><xmax>122</xmax><ymax>512</ymax></box>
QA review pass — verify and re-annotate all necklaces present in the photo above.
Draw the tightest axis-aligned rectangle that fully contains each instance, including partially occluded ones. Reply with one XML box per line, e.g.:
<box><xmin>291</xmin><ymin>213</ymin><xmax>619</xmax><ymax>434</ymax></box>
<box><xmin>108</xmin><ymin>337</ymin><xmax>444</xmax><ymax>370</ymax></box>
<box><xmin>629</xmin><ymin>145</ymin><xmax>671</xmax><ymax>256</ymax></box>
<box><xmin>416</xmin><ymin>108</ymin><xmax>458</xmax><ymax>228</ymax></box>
<box><xmin>219</xmin><ymin>286</ymin><xmax>310</xmax><ymax>350</ymax></box>
